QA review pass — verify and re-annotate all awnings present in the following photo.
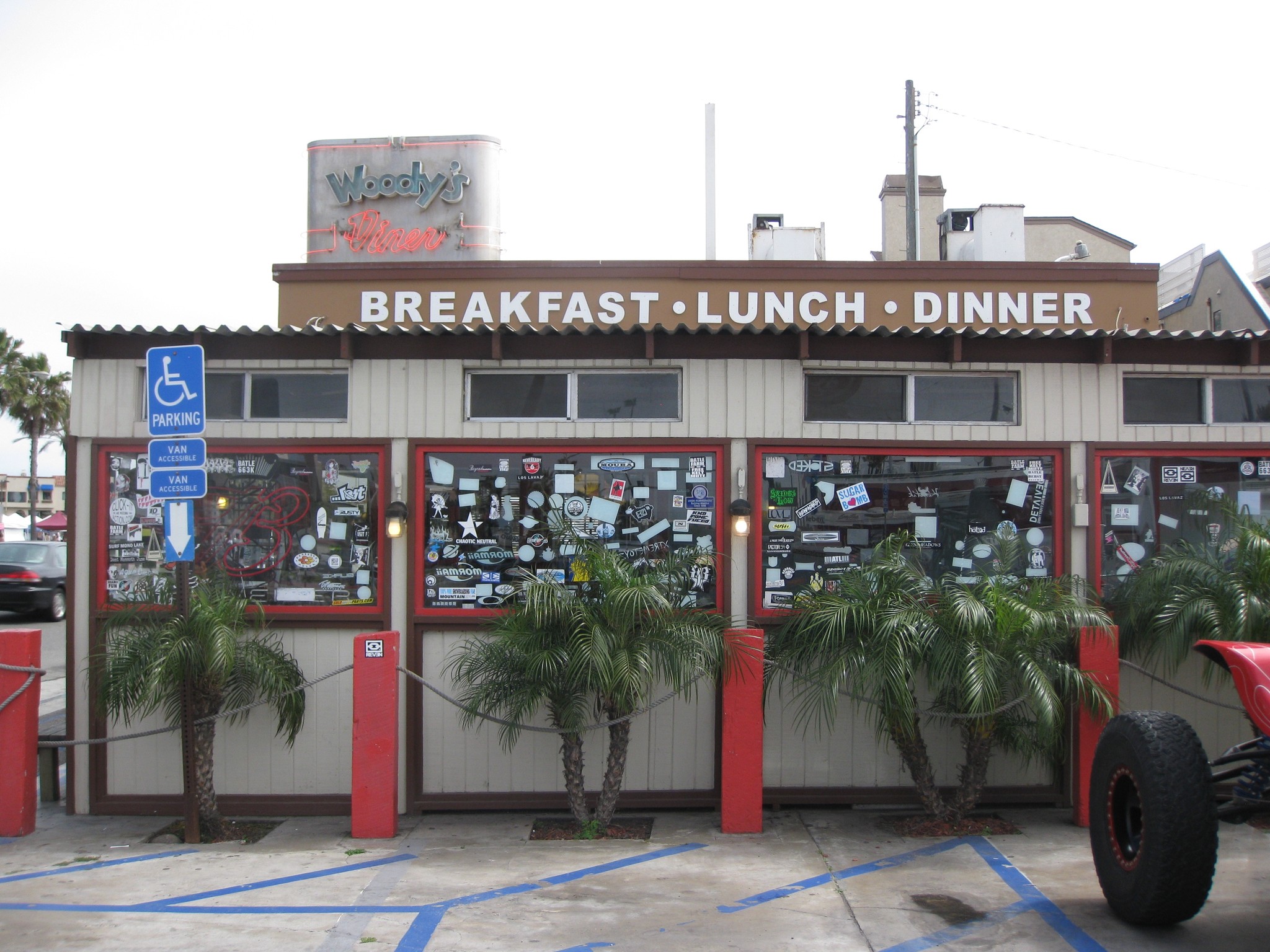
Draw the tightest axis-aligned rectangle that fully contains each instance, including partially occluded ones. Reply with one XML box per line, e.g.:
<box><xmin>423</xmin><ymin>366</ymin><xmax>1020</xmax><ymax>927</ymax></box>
<box><xmin>41</xmin><ymin>485</ymin><xmax>53</xmax><ymax>490</ymax></box>
<box><xmin>27</xmin><ymin>485</ymin><xmax>40</xmax><ymax>490</ymax></box>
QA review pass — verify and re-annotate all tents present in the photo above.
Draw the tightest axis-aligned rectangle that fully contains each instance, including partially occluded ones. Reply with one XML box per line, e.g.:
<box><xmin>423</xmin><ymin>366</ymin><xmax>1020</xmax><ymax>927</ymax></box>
<box><xmin>25</xmin><ymin>515</ymin><xmax>42</xmax><ymax>524</ymax></box>
<box><xmin>24</xmin><ymin>524</ymin><xmax>49</xmax><ymax>541</ymax></box>
<box><xmin>36</xmin><ymin>510</ymin><xmax>67</xmax><ymax>541</ymax></box>
<box><xmin>2</xmin><ymin>513</ymin><xmax>29</xmax><ymax>542</ymax></box>
<box><xmin>42</xmin><ymin>515</ymin><xmax>52</xmax><ymax>520</ymax></box>
<box><xmin>9</xmin><ymin>512</ymin><xmax>31</xmax><ymax>541</ymax></box>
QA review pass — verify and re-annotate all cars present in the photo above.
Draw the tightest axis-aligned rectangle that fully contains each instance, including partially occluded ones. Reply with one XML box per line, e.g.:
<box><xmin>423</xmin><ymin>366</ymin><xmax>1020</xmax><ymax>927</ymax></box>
<box><xmin>0</xmin><ymin>540</ymin><xmax>67</xmax><ymax>623</ymax></box>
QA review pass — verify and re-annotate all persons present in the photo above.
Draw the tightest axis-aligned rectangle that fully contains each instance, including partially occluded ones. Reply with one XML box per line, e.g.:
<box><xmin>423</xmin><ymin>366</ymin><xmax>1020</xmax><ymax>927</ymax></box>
<box><xmin>44</xmin><ymin>532</ymin><xmax>48</xmax><ymax>540</ymax></box>
<box><xmin>53</xmin><ymin>531</ymin><xmax>61</xmax><ymax>541</ymax></box>
